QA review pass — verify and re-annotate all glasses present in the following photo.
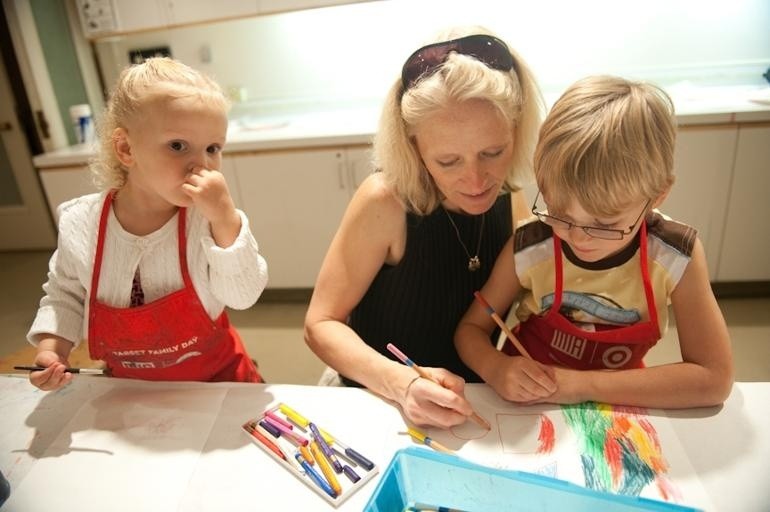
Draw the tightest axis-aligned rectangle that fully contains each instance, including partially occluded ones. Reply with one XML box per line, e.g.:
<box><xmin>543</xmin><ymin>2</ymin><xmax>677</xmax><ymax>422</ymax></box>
<box><xmin>531</xmin><ymin>189</ymin><xmax>651</xmax><ymax>240</ymax></box>
<box><xmin>402</xmin><ymin>34</ymin><xmax>517</xmax><ymax>92</ymax></box>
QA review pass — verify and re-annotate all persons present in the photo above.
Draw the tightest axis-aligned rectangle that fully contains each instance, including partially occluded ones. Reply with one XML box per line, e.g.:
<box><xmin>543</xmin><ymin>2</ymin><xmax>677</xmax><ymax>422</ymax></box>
<box><xmin>29</xmin><ymin>57</ymin><xmax>269</xmax><ymax>392</ymax></box>
<box><xmin>302</xmin><ymin>25</ymin><xmax>534</xmax><ymax>430</ymax></box>
<box><xmin>452</xmin><ymin>74</ymin><xmax>735</xmax><ymax>409</ymax></box>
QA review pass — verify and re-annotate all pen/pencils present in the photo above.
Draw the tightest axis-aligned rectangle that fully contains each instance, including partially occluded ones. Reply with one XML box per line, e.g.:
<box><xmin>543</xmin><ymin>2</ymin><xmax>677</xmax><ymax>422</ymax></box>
<box><xmin>387</xmin><ymin>344</ymin><xmax>492</xmax><ymax>431</ymax></box>
<box><xmin>245</xmin><ymin>405</ymin><xmax>373</xmax><ymax>498</ymax></box>
<box><xmin>405</xmin><ymin>503</ymin><xmax>468</xmax><ymax>512</ymax></box>
<box><xmin>408</xmin><ymin>427</ymin><xmax>456</xmax><ymax>455</ymax></box>
<box><xmin>474</xmin><ymin>291</ymin><xmax>534</xmax><ymax>361</ymax></box>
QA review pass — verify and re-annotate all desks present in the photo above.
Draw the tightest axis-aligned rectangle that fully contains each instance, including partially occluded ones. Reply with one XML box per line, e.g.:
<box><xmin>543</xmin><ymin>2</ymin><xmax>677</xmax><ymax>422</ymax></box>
<box><xmin>0</xmin><ymin>369</ymin><xmax>770</xmax><ymax>512</ymax></box>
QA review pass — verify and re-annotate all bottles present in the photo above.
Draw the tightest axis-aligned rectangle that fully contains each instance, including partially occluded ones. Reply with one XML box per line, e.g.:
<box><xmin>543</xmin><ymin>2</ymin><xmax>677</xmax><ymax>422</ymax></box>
<box><xmin>68</xmin><ymin>102</ymin><xmax>96</xmax><ymax>147</ymax></box>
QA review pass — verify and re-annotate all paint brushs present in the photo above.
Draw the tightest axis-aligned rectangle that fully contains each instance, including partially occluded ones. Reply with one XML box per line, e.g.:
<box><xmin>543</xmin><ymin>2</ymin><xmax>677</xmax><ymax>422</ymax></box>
<box><xmin>13</xmin><ymin>366</ymin><xmax>112</xmax><ymax>374</ymax></box>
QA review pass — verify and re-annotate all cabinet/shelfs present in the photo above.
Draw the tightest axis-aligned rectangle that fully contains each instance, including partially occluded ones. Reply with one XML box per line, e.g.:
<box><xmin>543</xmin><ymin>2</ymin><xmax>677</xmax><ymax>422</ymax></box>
<box><xmin>34</xmin><ymin>84</ymin><xmax>769</xmax><ymax>304</ymax></box>
<box><xmin>73</xmin><ymin>1</ymin><xmax>380</xmax><ymax>39</ymax></box>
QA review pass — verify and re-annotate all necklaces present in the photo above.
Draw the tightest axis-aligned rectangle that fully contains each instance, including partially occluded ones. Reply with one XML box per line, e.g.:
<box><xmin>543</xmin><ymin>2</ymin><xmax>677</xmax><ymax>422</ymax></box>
<box><xmin>440</xmin><ymin>199</ymin><xmax>487</xmax><ymax>273</ymax></box>
